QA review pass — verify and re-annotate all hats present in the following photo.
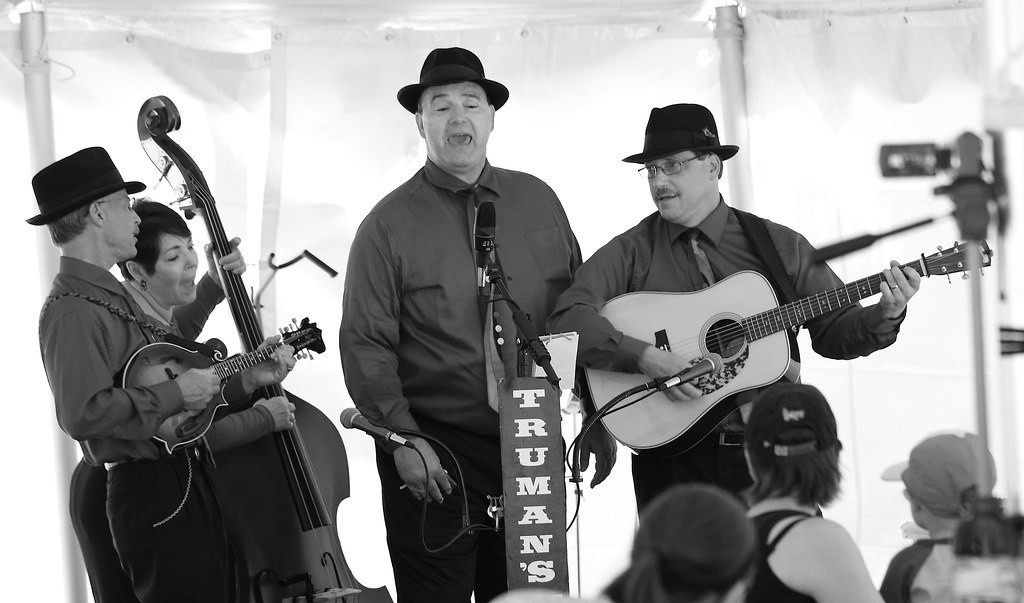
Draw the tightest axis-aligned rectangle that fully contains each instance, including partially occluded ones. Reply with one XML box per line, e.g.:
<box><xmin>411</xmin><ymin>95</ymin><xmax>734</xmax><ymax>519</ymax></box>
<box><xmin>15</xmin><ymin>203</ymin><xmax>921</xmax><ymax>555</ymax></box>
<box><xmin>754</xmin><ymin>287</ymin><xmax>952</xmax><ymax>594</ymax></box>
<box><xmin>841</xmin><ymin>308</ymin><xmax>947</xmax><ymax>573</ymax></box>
<box><xmin>27</xmin><ymin>146</ymin><xmax>146</xmax><ymax>226</ymax></box>
<box><xmin>622</xmin><ymin>104</ymin><xmax>739</xmax><ymax>163</ymax></box>
<box><xmin>397</xmin><ymin>47</ymin><xmax>509</xmax><ymax>114</ymax></box>
<box><xmin>882</xmin><ymin>433</ymin><xmax>996</xmax><ymax>513</ymax></box>
<box><xmin>747</xmin><ymin>383</ymin><xmax>837</xmax><ymax>458</ymax></box>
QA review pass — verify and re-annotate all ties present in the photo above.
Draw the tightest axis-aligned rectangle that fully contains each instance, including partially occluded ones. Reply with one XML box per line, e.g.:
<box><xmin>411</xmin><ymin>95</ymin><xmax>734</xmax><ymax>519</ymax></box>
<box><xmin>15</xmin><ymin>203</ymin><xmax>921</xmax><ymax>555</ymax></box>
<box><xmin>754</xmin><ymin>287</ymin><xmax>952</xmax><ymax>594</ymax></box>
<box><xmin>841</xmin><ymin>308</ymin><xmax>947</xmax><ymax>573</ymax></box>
<box><xmin>116</xmin><ymin>281</ymin><xmax>216</xmax><ymax>470</ymax></box>
<box><xmin>466</xmin><ymin>185</ymin><xmax>518</xmax><ymax>414</ymax></box>
<box><xmin>685</xmin><ymin>231</ymin><xmax>716</xmax><ymax>288</ymax></box>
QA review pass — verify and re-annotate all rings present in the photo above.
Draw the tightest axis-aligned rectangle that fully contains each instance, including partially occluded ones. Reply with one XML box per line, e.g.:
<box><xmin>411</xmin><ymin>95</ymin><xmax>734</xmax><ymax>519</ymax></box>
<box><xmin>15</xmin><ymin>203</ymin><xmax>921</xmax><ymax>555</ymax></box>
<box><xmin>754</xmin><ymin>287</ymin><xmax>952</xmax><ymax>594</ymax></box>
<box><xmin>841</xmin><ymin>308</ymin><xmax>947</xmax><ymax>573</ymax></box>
<box><xmin>287</xmin><ymin>363</ymin><xmax>295</xmax><ymax>371</ymax></box>
<box><xmin>891</xmin><ymin>286</ymin><xmax>899</xmax><ymax>289</ymax></box>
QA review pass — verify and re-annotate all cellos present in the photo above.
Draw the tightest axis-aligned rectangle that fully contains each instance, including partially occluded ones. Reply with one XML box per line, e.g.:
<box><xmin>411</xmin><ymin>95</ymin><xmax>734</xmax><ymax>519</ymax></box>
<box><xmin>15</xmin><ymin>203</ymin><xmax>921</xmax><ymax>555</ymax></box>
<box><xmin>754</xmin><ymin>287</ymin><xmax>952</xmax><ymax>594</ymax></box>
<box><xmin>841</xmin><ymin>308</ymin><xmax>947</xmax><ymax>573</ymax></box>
<box><xmin>137</xmin><ymin>94</ymin><xmax>395</xmax><ymax>602</ymax></box>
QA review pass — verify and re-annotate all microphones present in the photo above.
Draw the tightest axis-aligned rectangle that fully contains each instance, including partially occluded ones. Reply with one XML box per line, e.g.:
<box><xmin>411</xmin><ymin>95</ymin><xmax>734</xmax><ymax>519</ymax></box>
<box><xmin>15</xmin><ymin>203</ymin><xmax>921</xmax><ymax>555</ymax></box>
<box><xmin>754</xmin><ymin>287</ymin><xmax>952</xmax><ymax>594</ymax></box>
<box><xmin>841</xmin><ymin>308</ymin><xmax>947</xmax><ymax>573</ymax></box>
<box><xmin>340</xmin><ymin>408</ymin><xmax>417</xmax><ymax>449</ymax></box>
<box><xmin>475</xmin><ymin>201</ymin><xmax>496</xmax><ymax>268</ymax></box>
<box><xmin>658</xmin><ymin>352</ymin><xmax>724</xmax><ymax>391</ymax></box>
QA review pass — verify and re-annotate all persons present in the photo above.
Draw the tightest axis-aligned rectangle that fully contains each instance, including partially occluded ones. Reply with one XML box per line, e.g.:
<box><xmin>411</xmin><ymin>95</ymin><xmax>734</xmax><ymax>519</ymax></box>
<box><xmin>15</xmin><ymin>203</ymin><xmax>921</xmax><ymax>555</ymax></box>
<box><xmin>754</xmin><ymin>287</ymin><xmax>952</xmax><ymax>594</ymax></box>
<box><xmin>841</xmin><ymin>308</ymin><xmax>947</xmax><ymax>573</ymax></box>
<box><xmin>541</xmin><ymin>104</ymin><xmax>923</xmax><ymax>524</ymax></box>
<box><xmin>488</xmin><ymin>483</ymin><xmax>758</xmax><ymax>603</ymax></box>
<box><xmin>115</xmin><ymin>198</ymin><xmax>297</xmax><ymax>464</ymax></box>
<box><xmin>25</xmin><ymin>143</ymin><xmax>296</xmax><ymax>603</ymax></box>
<box><xmin>338</xmin><ymin>45</ymin><xmax>616</xmax><ymax>603</ymax></box>
<box><xmin>734</xmin><ymin>382</ymin><xmax>888</xmax><ymax>603</ymax></box>
<box><xmin>880</xmin><ymin>434</ymin><xmax>1024</xmax><ymax>603</ymax></box>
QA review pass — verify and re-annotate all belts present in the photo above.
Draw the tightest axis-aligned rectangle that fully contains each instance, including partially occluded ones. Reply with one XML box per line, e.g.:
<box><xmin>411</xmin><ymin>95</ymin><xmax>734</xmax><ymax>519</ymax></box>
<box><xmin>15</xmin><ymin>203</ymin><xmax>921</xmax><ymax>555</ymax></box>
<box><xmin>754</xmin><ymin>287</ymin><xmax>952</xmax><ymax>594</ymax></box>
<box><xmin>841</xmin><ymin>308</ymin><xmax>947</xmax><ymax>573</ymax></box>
<box><xmin>132</xmin><ymin>447</ymin><xmax>204</xmax><ymax>463</ymax></box>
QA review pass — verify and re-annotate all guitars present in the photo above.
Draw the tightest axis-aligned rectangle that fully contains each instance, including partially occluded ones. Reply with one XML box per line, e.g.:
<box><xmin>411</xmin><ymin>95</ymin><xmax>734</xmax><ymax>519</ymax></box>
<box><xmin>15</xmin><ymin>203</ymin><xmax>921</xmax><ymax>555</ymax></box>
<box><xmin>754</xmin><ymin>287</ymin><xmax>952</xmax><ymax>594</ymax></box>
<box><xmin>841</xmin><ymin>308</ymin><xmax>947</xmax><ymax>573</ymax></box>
<box><xmin>120</xmin><ymin>315</ymin><xmax>327</xmax><ymax>456</ymax></box>
<box><xmin>583</xmin><ymin>239</ymin><xmax>992</xmax><ymax>464</ymax></box>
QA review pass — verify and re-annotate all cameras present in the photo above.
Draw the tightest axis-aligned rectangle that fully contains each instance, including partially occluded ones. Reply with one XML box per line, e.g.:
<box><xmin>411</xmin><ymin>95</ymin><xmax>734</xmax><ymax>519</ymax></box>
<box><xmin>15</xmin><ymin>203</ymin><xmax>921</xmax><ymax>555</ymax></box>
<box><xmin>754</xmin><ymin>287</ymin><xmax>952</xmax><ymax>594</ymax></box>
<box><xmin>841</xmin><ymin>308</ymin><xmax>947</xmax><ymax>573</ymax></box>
<box><xmin>879</xmin><ymin>133</ymin><xmax>983</xmax><ymax>180</ymax></box>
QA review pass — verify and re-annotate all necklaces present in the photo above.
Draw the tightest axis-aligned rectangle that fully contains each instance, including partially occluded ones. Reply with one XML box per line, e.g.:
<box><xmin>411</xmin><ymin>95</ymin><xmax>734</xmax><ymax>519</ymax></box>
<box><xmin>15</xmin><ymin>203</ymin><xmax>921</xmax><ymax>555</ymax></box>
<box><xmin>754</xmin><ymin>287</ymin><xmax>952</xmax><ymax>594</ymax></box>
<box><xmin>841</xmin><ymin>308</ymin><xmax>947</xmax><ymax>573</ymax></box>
<box><xmin>131</xmin><ymin>286</ymin><xmax>174</xmax><ymax>326</ymax></box>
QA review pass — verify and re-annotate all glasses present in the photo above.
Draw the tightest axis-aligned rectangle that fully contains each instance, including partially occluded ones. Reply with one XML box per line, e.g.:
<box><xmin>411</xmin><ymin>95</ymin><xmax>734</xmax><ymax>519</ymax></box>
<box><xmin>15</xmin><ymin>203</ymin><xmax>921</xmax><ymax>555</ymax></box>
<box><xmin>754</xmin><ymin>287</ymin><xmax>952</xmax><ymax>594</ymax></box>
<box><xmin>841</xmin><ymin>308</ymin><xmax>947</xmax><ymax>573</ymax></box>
<box><xmin>638</xmin><ymin>153</ymin><xmax>708</xmax><ymax>178</ymax></box>
<box><xmin>83</xmin><ymin>197</ymin><xmax>135</xmax><ymax>217</ymax></box>
